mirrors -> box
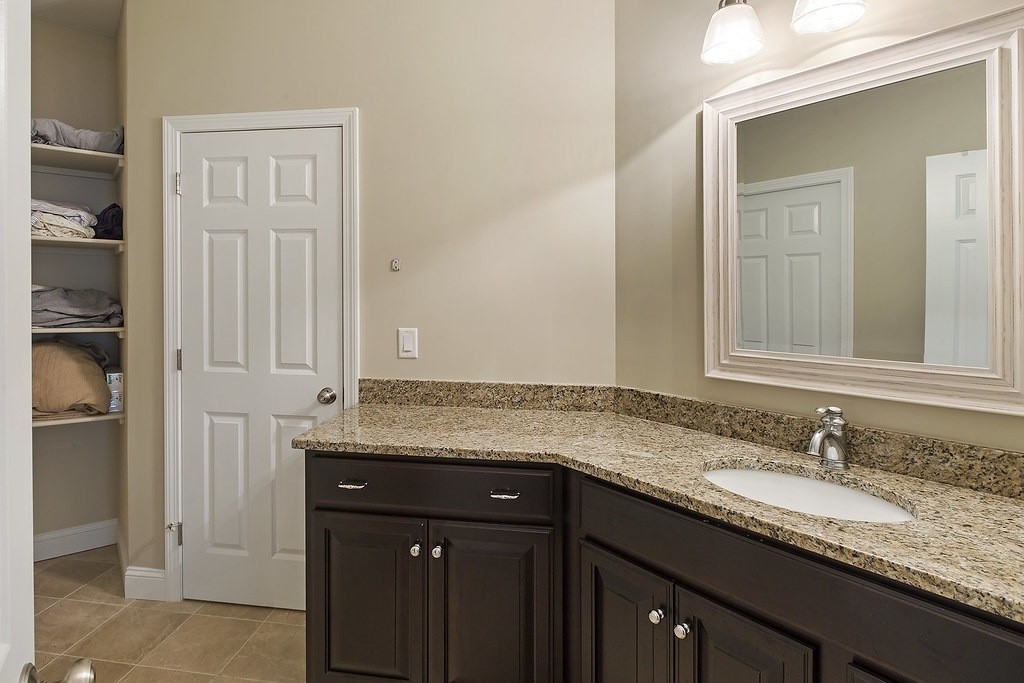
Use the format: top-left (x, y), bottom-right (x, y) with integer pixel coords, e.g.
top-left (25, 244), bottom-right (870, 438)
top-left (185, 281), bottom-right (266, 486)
top-left (702, 2), bottom-right (1024, 418)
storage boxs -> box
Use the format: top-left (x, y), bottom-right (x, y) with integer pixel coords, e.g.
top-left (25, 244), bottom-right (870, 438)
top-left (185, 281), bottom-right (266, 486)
top-left (108, 392), bottom-right (124, 412)
top-left (104, 367), bottom-right (124, 392)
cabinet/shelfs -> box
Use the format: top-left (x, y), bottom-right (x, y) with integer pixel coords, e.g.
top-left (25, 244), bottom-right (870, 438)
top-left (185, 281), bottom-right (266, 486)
top-left (32, 142), bottom-right (125, 428)
top-left (566, 470), bottom-right (1024, 683)
top-left (305, 449), bottom-right (567, 683)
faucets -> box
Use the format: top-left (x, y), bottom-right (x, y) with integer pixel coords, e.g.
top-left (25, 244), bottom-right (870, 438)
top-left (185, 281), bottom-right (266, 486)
top-left (808, 404), bottom-right (848, 471)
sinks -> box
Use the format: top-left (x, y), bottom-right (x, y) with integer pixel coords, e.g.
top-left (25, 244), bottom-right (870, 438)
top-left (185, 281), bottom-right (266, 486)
top-left (700, 460), bottom-right (919, 523)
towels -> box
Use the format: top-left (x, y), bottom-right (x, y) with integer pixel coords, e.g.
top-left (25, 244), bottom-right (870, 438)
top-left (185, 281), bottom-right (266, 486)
top-left (31, 199), bottom-right (98, 239)
top-left (31, 284), bottom-right (125, 329)
top-left (96, 203), bottom-right (122, 239)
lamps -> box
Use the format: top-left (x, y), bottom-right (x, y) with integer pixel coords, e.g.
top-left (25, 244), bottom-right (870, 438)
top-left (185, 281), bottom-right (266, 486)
top-left (700, 0), bottom-right (870, 66)
